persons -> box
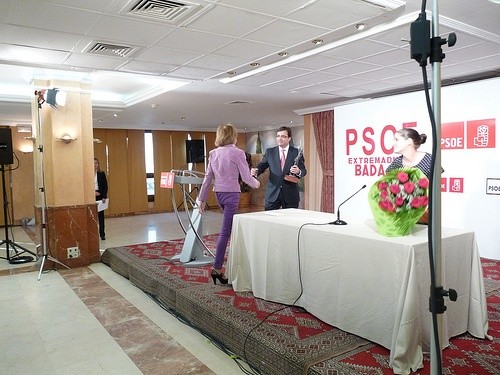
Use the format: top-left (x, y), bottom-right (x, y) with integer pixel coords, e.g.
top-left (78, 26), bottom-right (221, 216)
top-left (384, 129), bottom-right (445, 224)
top-left (198, 124), bottom-right (260, 285)
top-left (251, 126), bottom-right (307, 211)
top-left (94, 157), bottom-right (108, 240)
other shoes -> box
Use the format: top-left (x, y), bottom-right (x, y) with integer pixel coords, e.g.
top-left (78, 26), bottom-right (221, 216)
top-left (101, 236), bottom-right (105, 240)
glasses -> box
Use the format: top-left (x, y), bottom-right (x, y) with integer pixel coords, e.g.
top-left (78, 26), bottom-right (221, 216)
top-left (276, 135), bottom-right (288, 139)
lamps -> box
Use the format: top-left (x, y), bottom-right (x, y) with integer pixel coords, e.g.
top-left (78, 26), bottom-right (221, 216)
top-left (46, 88), bottom-right (66, 107)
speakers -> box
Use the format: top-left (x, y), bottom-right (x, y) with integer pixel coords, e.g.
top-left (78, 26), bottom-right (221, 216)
top-left (0, 127), bottom-right (13, 164)
top-left (186, 139), bottom-right (204, 163)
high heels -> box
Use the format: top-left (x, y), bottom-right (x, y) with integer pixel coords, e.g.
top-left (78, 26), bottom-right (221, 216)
top-left (211, 269), bottom-right (228, 285)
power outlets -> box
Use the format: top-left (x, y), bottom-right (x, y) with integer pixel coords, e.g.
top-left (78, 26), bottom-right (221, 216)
top-left (67, 247), bottom-right (78, 259)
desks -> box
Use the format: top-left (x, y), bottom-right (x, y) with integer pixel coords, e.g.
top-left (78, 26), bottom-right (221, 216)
top-left (223, 208), bottom-right (492, 375)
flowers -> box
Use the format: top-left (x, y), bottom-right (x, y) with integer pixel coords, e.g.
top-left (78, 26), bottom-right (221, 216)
top-left (377, 173), bottom-right (428, 212)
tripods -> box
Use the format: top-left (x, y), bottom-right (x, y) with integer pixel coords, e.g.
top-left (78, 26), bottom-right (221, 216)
top-left (0, 164), bottom-right (36, 260)
top-left (31, 106), bottom-right (70, 280)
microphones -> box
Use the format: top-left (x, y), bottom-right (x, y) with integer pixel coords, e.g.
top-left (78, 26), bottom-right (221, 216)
top-left (333, 185), bottom-right (366, 225)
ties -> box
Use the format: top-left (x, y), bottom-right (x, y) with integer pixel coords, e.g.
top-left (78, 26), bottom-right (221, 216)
top-left (280, 150), bottom-right (286, 172)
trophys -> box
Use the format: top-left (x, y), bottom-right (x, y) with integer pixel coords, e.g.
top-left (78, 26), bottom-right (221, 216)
top-left (284, 141), bottom-right (303, 183)
